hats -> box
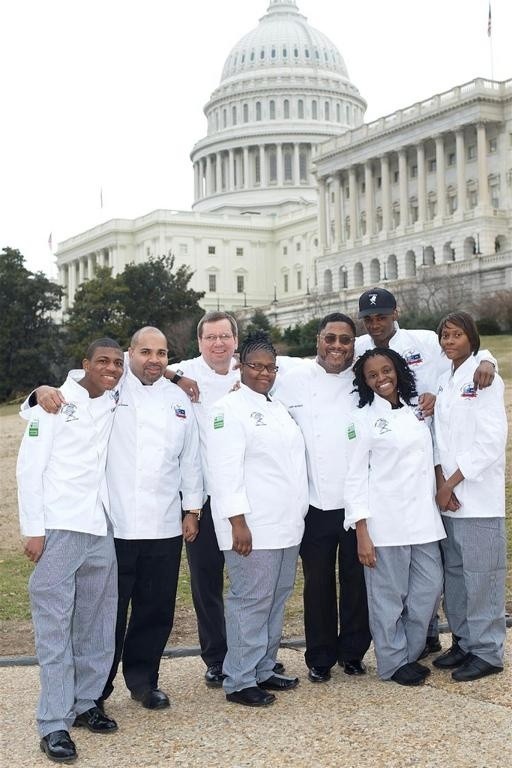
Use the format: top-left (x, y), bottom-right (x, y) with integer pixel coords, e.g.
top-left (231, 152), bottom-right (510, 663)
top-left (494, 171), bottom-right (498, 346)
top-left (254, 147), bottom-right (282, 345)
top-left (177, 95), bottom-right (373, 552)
top-left (357, 287), bottom-right (397, 319)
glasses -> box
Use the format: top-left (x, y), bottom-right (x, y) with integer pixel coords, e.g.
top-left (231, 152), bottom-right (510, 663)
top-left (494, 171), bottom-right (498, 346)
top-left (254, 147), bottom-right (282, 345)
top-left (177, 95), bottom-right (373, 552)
top-left (321, 334), bottom-right (355, 346)
top-left (200, 333), bottom-right (232, 340)
top-left (243, 362), bottom-right (279, 374)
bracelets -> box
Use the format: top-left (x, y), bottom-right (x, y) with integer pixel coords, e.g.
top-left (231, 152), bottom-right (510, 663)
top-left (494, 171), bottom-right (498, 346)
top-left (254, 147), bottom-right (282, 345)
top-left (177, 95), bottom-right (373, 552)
top-left (185, 509), bottom-right (204, 521)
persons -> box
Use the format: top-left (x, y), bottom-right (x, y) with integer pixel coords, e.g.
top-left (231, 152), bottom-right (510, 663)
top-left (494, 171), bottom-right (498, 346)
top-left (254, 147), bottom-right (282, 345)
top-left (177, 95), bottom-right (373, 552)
top-left (166, 311), bottom-right (284, 687)
top-left (341, 346), bottom-right (447, 687)
top-left (230, 312), bottom-right (437, 683)
top-left (15, 337), bottom-right (200, 762)
top-left (199, 337), bottom-right (309, 707)
top-left (353, 287), bottom-right (498, 661)
top-left (428, 310), bottom-right (508, 683)
top-left (20, 326), bottom-right (207, 710)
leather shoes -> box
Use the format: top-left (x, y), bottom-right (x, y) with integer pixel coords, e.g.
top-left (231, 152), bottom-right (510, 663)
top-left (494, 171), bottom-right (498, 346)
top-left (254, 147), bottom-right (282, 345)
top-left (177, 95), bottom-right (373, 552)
top-left (40, 730), bottom-right (78, 762)
top-left (72, 705), bottom-right (117, 735)
top-left (130, 688), bottom-right (171, 711)
top-left (391, 635), bottom-right (504, 686)
top-left (205, 662), bottom-right (299, 707)
top-left (338, 660), bottom-right (368, 677)
top-left (307, 667), bottom-right (335, 683)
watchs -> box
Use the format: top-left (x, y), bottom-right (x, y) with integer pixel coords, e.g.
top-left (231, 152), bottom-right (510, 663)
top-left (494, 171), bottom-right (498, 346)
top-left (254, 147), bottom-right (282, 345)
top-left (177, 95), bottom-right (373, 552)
top-left (170, 369), bottom-right (184, 383)
